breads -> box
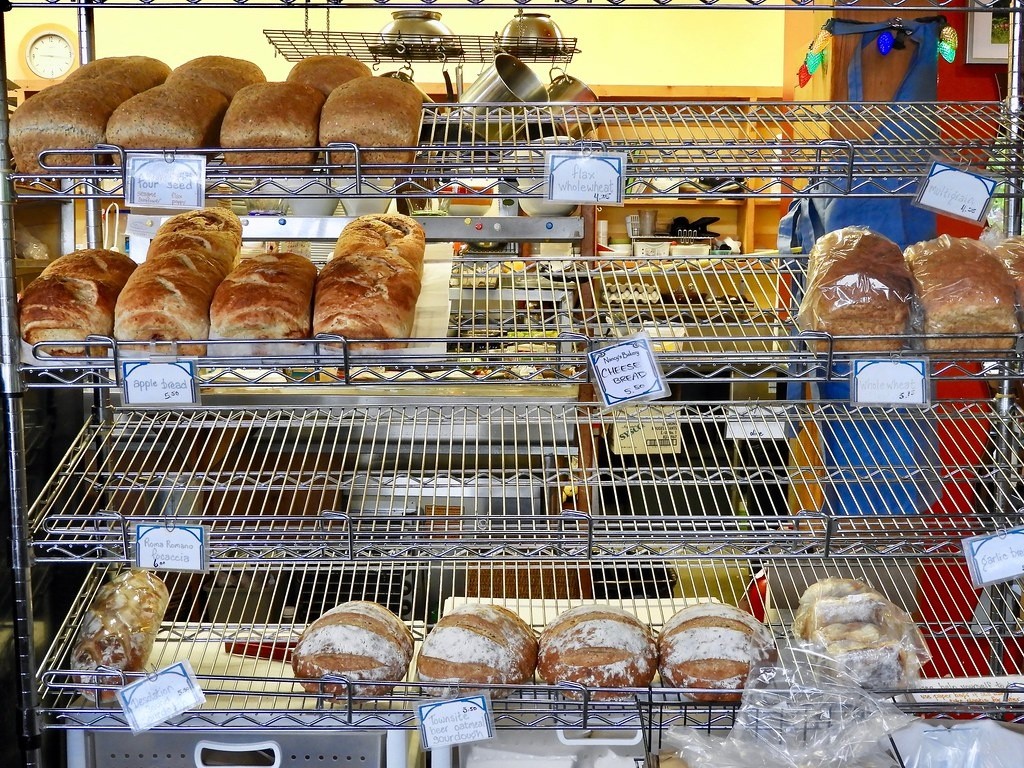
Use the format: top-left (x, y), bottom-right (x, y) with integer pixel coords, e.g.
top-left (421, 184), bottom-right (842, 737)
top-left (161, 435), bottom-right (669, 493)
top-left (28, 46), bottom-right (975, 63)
top-left (73, 568), bottom-right (171, 702)
top-left (19, 208), bottom-right (426, 364)
top-left (803, 226), bottom-right (1023, 350)
top-left (798, 581), bottom-right (916, 688)
top-left (292, 599), bottom-right (537, 700)
top-left (7, 56), bottom-right (422, 178)
top-left (538, 603), bottom-right (774, 702)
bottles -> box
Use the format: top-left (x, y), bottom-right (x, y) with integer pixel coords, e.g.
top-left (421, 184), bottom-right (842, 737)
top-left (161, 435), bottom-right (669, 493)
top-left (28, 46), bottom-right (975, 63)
top-left (217, 186), bottom-right (231, 210)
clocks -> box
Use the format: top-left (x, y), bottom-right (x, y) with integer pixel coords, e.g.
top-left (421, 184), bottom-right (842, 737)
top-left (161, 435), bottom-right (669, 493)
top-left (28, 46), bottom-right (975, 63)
top-left (18, 23), bottom-right (80, 79)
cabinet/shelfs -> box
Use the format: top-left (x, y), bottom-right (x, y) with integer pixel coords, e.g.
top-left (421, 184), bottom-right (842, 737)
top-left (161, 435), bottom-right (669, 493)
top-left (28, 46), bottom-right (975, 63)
top-left (0, 0), bottom-right (1024, 768)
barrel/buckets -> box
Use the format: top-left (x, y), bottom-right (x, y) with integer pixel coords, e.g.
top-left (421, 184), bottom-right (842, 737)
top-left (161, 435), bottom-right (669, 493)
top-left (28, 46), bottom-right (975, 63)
top-left (522, 67), bottom-right (603, 142)
top-left (458, 48), bottom-right (550, 142)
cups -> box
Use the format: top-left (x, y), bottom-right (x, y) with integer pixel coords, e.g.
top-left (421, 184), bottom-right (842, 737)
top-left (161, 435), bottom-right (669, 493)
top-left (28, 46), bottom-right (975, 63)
top-left (597, 220), bottom-right (608, 247)
top-left (638, 210), bottom-right (657, 236)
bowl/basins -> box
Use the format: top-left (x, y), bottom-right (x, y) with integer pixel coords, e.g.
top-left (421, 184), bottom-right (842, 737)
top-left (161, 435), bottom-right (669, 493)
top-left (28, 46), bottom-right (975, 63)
top-left (310, 243), bottom-right (338, 270)
top-left (240, 241), bottom-right (264, 258)
top-left (518, 197), bottom-right (578, 217)
top-left (437, 186), bottom-right (493, 216)
top-left (241, 192), bottom-right (289, 216)
top-left (498, 14), bottom-right (564, 57)
top-left (286, 188), bottom-right (340, 216)
top-left (368, 10), bottom-right (461, 60)
top-left (335, 186), bottom-right (395, 217)
top-left (402, 191), bottom-right (453, 217)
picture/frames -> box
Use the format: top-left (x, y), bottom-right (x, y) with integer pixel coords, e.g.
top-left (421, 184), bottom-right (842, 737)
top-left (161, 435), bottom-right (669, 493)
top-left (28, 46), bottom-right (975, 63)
top-left (966, 0), bottom-right (1012, 64)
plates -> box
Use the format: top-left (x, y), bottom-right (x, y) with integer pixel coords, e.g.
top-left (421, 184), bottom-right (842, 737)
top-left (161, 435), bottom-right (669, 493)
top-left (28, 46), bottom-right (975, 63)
top-left (599, 251), bottom-right (630, 258)
